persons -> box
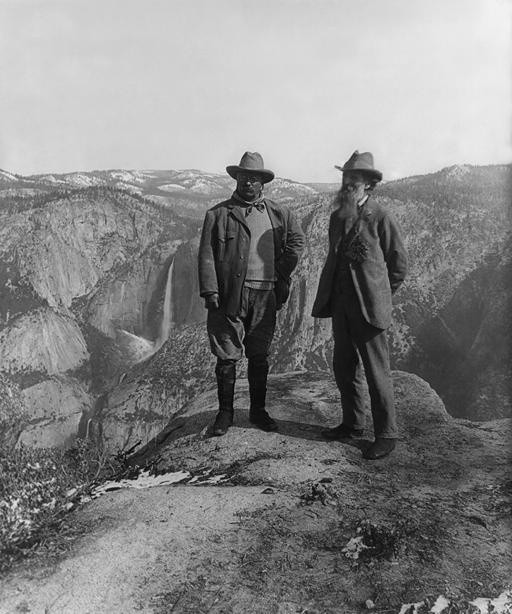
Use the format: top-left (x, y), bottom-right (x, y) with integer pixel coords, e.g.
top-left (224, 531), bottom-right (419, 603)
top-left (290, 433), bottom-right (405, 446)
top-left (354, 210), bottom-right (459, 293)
top-left (310, 150), bottom-right (409, 460)
top-left (198, 152), bottom-right (306, 436)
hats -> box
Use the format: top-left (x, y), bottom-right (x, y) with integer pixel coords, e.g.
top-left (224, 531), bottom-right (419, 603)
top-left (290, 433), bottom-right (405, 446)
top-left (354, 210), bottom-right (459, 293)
top-left (225, 152), bottom-right (274, 183)
top-left (334, 150), bottom-right (382, 183)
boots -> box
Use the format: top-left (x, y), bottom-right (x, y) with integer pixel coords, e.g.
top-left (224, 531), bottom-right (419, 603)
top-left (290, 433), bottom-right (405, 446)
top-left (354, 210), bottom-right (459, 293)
top-left (213, 364), bottom-right (236, 436)
top-left (247, 364), bottom-right (278, 431)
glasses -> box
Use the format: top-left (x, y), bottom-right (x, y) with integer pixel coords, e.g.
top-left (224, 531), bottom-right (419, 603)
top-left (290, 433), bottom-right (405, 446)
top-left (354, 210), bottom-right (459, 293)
top-left (234, 174), bottom-right (258, 183)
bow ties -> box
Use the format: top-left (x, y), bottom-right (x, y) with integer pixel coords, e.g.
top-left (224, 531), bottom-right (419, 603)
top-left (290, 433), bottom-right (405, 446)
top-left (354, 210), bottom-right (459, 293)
top-left (246, 203), bottom-right (264, 215)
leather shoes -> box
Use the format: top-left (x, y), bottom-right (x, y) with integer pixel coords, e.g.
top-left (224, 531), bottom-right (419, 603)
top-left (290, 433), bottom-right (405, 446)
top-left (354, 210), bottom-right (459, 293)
top-left (321, 423), bottom-right (366, 439)
top-left (362, 439), bottom-right (395, 459)
